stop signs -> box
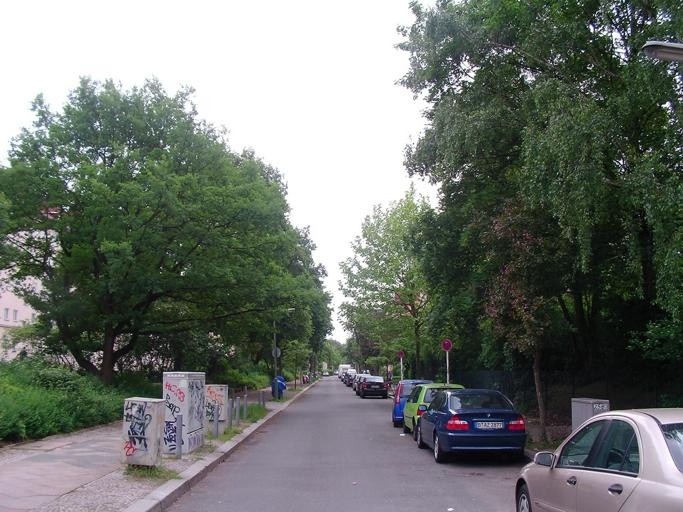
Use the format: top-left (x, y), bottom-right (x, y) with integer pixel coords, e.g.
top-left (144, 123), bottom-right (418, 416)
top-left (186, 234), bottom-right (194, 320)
top-left (442, 339), bottom-right (452, 352)
top-left (399, 351), bottom-right (404, 358)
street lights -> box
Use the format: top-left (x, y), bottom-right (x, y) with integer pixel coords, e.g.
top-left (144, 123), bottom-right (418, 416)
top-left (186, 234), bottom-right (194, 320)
top-left (273, 307), bottom-right (296, 401)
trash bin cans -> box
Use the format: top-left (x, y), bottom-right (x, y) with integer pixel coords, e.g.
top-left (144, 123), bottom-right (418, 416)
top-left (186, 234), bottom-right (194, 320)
top-left (272, 376), bottom-right (286, 399)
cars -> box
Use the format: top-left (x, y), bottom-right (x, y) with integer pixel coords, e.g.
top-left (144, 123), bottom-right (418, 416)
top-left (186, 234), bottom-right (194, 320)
top-left (337, 363), bottom-right (389, 399)
top-left (390, 378), bottom-right (530, 462)
top-left (511, 404), bottom-right (683, 512)
